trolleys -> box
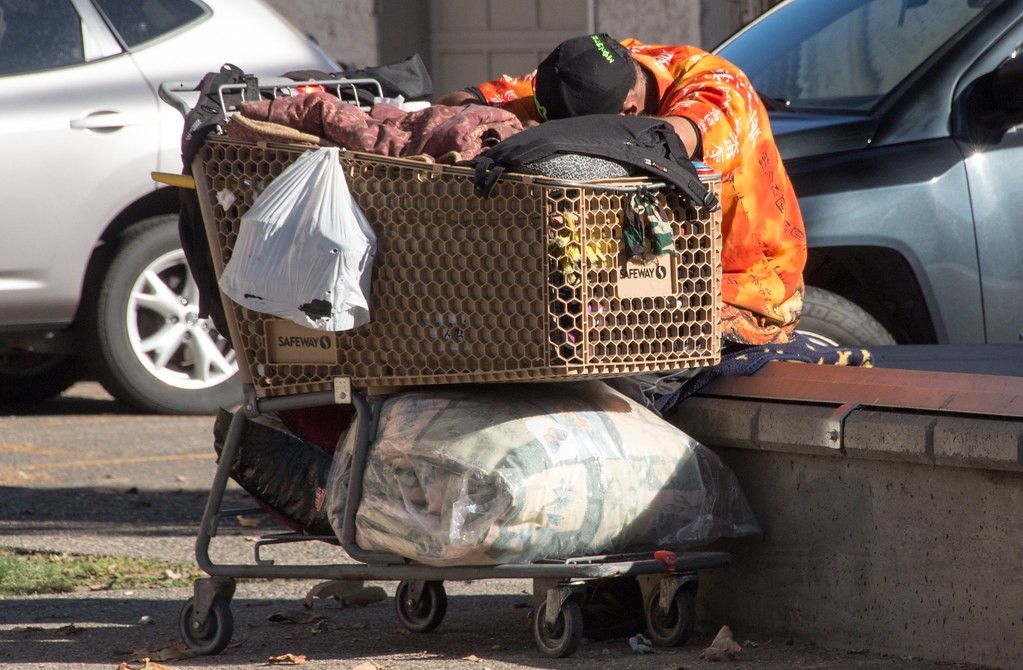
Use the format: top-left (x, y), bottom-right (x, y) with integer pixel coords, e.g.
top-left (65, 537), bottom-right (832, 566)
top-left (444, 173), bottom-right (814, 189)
top-left (157, 67), bottom-right (740, 660)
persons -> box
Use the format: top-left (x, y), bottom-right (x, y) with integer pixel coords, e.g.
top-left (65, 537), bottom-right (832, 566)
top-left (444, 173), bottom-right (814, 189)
top-left (440, 34), bottom-right (809, 628)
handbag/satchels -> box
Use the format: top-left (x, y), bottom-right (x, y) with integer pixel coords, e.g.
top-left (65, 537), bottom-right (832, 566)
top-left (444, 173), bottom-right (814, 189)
top-left (217, 147), bottom-right (376, 332)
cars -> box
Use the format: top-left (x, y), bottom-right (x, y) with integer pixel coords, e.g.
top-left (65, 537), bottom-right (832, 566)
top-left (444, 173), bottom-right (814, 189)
top-left (704, 0), bottom-right (1023, 356)
top-left (0, 0), bottom-right (353, 419)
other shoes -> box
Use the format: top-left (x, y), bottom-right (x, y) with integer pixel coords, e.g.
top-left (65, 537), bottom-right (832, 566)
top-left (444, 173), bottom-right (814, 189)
top-left (556, 575), bottom-right (647, 639)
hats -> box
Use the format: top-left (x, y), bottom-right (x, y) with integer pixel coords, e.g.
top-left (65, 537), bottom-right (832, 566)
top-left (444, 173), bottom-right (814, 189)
top-left (531, 34), bottom-right (635, 124)
